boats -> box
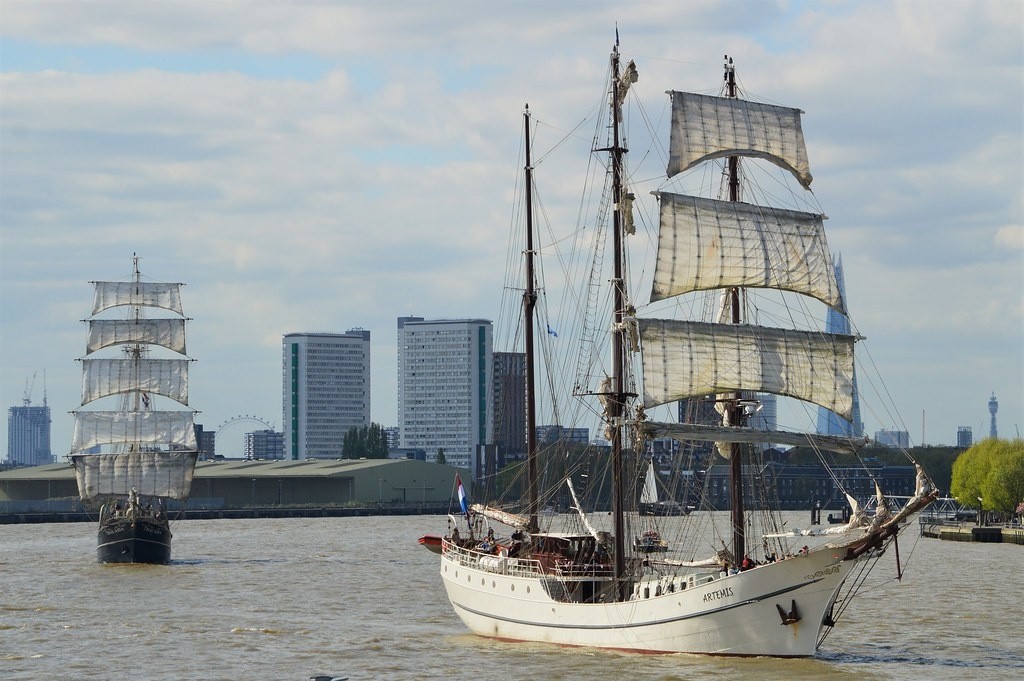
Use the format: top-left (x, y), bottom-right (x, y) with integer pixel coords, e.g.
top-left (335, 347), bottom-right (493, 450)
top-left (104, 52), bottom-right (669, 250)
top-left (418, 535), bottom-right (483, 556)
top-left (632, 529), bottom-right (669, 554)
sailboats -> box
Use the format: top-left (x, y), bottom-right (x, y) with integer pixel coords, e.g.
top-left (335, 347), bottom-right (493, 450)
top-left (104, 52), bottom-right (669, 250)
top-left (637, 457), bottom-right (695, 516)
top-left (439, 21), bottom-right (942, 660)
top-left (59, 252), bottom-right (209, 570)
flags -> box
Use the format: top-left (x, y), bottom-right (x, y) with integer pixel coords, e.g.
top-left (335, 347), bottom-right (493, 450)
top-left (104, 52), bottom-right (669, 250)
top-left (463, 485), bottom-right (464, 486)
top-left (548, 324), bottom-right (558, 337)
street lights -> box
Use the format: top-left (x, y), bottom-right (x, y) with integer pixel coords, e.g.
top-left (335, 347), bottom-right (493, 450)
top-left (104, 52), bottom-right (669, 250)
top-left (979, 498), bottom-right (983, 528)
top-left (955, 496), bottom-right (959, 527)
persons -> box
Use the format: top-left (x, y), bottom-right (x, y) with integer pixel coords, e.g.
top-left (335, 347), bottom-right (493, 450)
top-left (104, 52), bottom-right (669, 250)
top-left (488, 526), bottom-right (494, 540)
top-left (455, 474), bottom-right (470, 516)
top-left (722, 553), bottom-right (755, 576)
top-left (452, 528), bottom-right (459, 541)
top-left (765, 552), bottom-right (775, 563)
